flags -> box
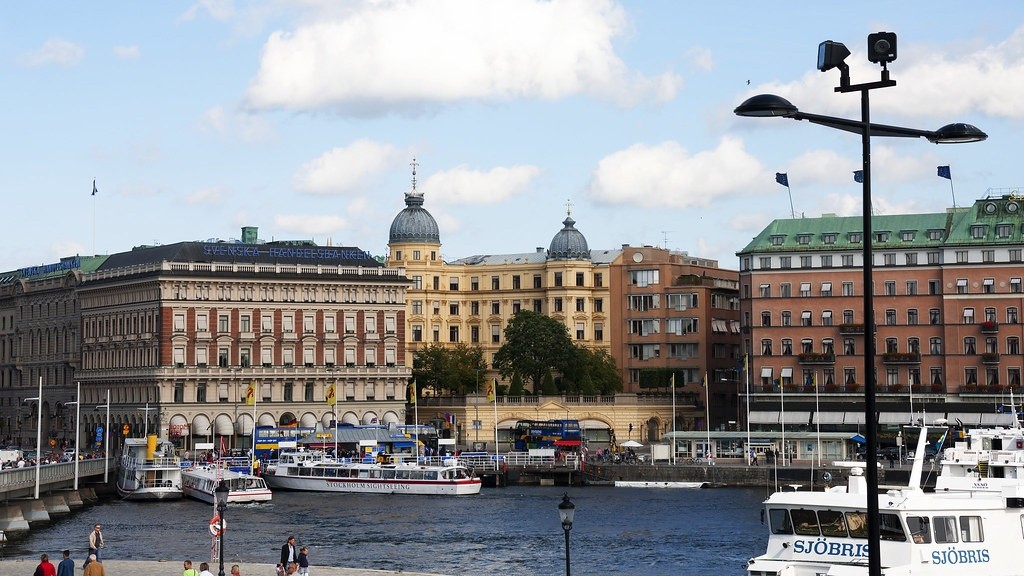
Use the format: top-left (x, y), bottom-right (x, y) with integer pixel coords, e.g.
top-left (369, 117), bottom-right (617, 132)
top-left (937, 166), bottom-right (950, 179)
top-left (328, 382), bottom-right (336, 405)
top-left (92, 181), bottom-right (97, 195)
top-left (775, 174), bottom-right (789, 188)
top-left (409, 382), bottom-right (415, 404)
top-left (486, 381), bottom-right (495, 402)
top-left (246, 382), bottom-right (255, 405)
top-left (854, 170), bottom-right (863, 182)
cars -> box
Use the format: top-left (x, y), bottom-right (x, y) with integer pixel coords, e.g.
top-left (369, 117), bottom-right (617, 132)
top-left (856, 446), bottom-right (905, 460)
top-left (63, 448), bottom-right (83, 456)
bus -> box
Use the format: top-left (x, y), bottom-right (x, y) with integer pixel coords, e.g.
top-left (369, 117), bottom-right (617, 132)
top-left (252, 425), bottom-right (316, 458)
top-left (510, 419), bottom-right (581, 455)
top-left (323, 423), bottom-right (439, 462)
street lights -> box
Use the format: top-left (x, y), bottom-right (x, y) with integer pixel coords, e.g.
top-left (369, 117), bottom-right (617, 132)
top-left (96, 390), bottom-right (110, 483)
top-left (733, 31), bottom-right (989, 576)
top-left (24, 376), bottom-right (43, 499)
top-left (214, 478), bottom-right (230, 576)
top-left (64, 382), bottom-right (80, 490)
top-left (558, 491), bottom-right (575, 576)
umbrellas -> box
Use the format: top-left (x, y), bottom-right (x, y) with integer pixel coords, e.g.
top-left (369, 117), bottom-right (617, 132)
top-left (618, 441), bottom-right (644, 449)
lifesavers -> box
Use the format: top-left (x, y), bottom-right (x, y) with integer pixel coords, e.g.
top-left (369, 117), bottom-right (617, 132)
top-left (210, 517), bottom-right (227, 536)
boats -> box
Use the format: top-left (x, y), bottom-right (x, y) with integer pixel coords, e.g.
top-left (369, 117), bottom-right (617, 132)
top-left (117, 403), bottom-right (184, 500)
top-left (933, 387), bottom-right (1024, 492)
top-left (260, 450), bottom-right (482, 494)
top-left (182, 463), bottom-right (272, 504)
top-left (747, 409), bottom-right (1024, 576)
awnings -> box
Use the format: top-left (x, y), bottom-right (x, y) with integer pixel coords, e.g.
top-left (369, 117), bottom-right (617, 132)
top-left (760, 283), bottom-right (832, 378)
top-left (958, 278), bottom-right (1021, 317)
top-left (748, 411), bottom-right (1014, 432)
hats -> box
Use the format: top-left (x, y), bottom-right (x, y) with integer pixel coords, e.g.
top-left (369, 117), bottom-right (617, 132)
top-left (89, 554), bottom-right (96, 560)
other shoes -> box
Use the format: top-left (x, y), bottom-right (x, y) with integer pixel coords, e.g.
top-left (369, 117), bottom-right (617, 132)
top-left (83, 564), bottom-right (86, 569)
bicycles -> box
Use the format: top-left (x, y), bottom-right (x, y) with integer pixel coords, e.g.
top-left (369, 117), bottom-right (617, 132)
top-left (686, 456), bottom-right (703, 465)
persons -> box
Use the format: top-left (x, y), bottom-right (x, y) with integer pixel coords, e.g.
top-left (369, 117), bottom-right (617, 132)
top-left (456, 448), bottom-right (469, 456)
top-left (889, 454), bottom-right (894, 468)
top-left (83, 524), bottom-right (104, 568)
top-left (199, 445), bottom-right (232, 461)
top-left (276, 537), bottom-right (309, 576)
top-left (751, 447), bottom-right (779, 466)
top-left (558, 447), bottom-right (609, 464)
top-left (425, 447), bottom-right (446, 456)
top-left (198, 563), bottom-right (214, 576)
top-left (248, 447), bottom-right (267, 475)
top-left (57, 550), bottom-right (74, 576)
top-left (83, 554), bottom-right (105, 576)
top-left (231, 566), bottom-right (240, 576)
top-left (183, 560), bottom-right (198, 576)
top-left (0, 450), bottom-right (106, 471)
top-left (33, 554), bottom-right (56, 576)
top-left (327, 448), bottom-right (364, 462)
top-left (183, 450), bottom-right (189, 461)
